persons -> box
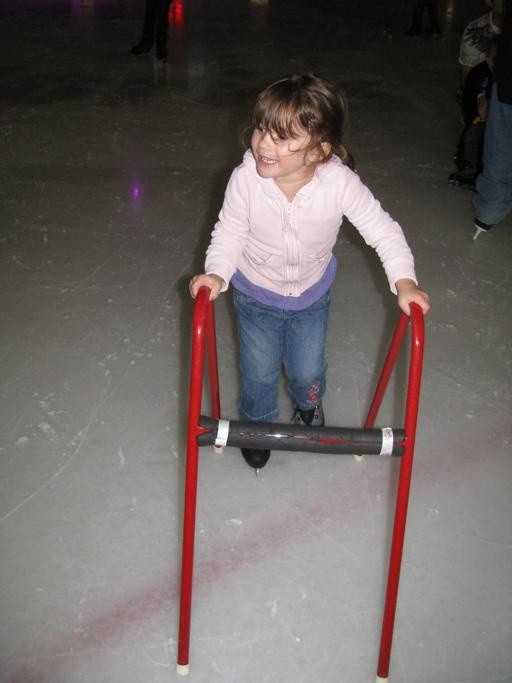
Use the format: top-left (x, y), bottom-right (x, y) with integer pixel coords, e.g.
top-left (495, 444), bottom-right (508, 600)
top-left (458, 13), bottom-right (509, 243)
top-left (188, 74), bottom-right (431, 469)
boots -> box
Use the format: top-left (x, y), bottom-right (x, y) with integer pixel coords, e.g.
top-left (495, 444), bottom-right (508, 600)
top-left (291, 401), bottom-right (324, 426)
top-left (242, 449), bottom-right (271, 468)
top-left (406, 26), bottom-right (440, 36)
top-left (475, 219), bottom-right (494, 232)
top-left (449, 172), bottom-right (475, 192)
top-left (132, 38), bottom-right (167, 59)
